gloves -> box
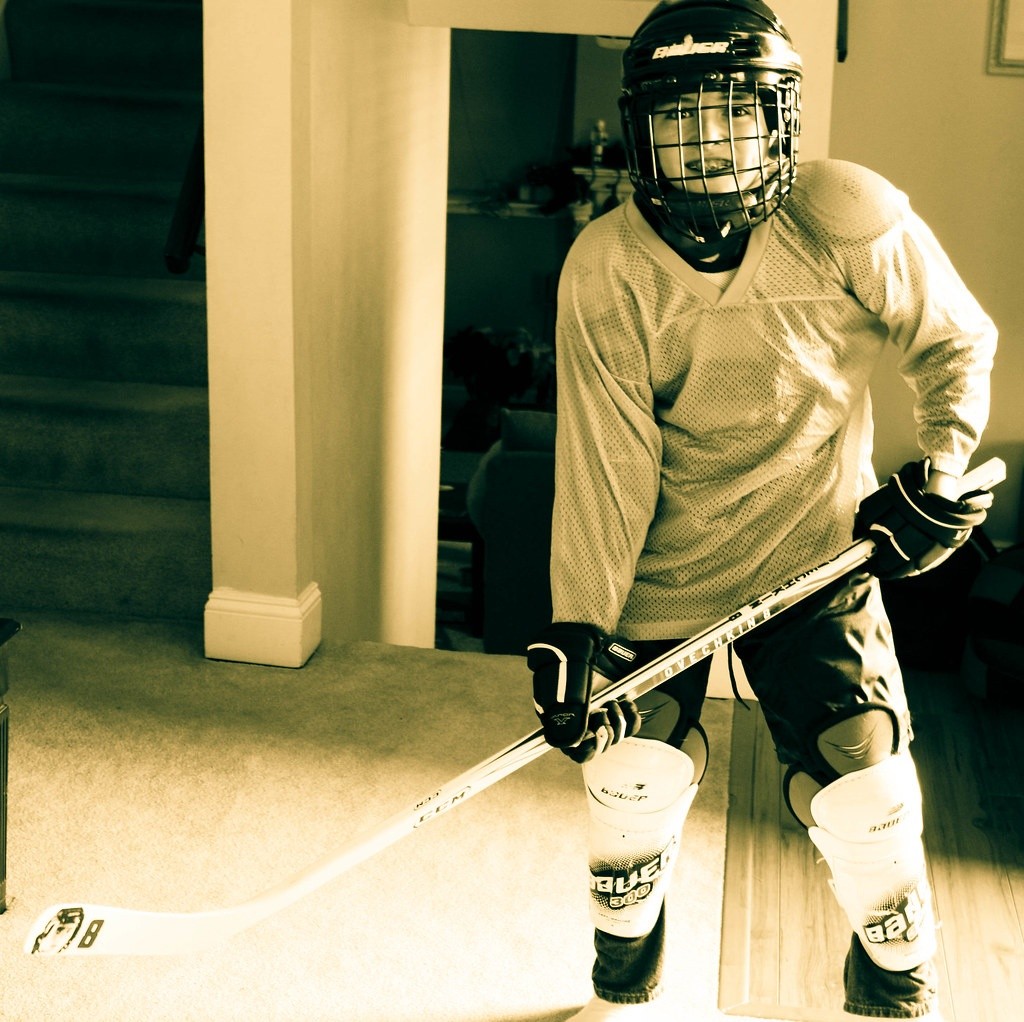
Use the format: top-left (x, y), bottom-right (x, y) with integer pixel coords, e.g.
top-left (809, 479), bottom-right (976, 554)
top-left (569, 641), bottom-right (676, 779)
top-left (852, 458), bottom-right (995, 579)
top-left (526, 622), bottom-right (642, 765)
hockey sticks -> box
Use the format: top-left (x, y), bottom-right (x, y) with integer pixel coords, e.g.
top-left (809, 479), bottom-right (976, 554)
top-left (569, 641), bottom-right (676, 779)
top-left (22, 454), bottom-right (1008, 966)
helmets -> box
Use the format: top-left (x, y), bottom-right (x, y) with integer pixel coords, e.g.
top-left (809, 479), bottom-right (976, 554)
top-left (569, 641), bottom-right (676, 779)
top-left (618, 0), bottom-right (804, 243)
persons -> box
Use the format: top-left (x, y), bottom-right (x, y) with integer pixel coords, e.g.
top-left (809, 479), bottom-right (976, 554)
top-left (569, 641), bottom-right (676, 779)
top-left (526, 0), bottom-right (999, 1022)
top-left (590, 120), bottom-right (609, 163)
